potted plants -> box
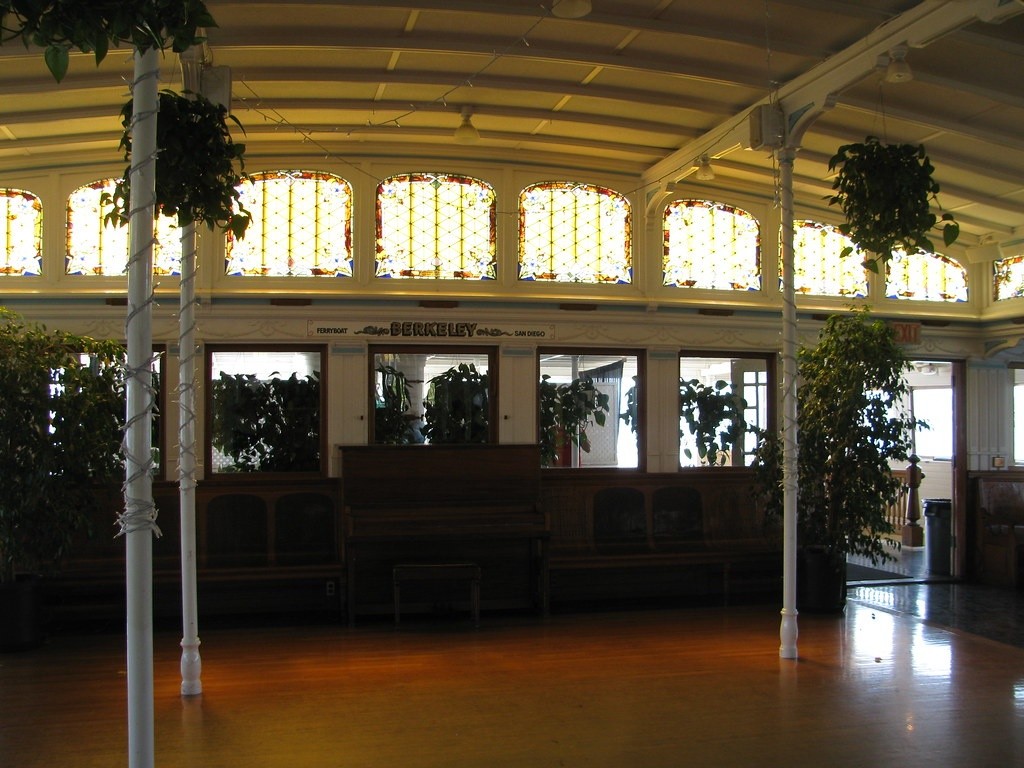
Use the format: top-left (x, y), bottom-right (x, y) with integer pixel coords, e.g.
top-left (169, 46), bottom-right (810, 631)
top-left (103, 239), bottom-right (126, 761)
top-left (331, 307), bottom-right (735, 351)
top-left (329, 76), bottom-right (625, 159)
top-left (679, 377), bottom-right (748, 465)
top-left (741, 309), bottom-right (931, 620)
top-left (821, 134), bottom-right (960, 276)
top-left (0, 0), bottom-right (222, 86)
top-left (421, 363), bottom-right (490, 446)
top-left (539, 375), bottom-right (611, 467)
top-left (373, 360), bottom-right (424, 446)
top-left (211, 370), bottom-right (270, 473)
top-left (99, 89), bottom-right (252, 239)
top-left (271, 371), bottom-right (321, 472)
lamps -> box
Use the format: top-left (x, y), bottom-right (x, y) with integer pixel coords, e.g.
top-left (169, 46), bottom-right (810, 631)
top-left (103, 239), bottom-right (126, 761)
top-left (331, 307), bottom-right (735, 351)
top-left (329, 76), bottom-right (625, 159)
top-left (888, 45), bottom-right (913, 85)
top-left (453, 106), bottom-right (480, 144)
top-left (553, 0), bottom-right (593, 19)
top-left (697, 155), bottom-right (715, 181)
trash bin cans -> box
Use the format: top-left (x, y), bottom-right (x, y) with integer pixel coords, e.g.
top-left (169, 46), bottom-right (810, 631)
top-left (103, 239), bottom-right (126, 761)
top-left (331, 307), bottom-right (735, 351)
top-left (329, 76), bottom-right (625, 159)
top-left (924, 498), bottom-right (951, 573)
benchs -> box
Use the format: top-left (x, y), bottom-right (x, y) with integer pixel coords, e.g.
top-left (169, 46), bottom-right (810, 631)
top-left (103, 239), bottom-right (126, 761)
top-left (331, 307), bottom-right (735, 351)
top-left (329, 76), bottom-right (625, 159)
top-left (974, 475), bottom-right (1024, 588)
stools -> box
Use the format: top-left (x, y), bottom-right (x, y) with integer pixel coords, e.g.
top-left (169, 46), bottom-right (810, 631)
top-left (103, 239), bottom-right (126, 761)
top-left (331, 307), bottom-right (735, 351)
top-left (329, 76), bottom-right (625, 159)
top-left (386, 558), bottom-right (480, 630)
